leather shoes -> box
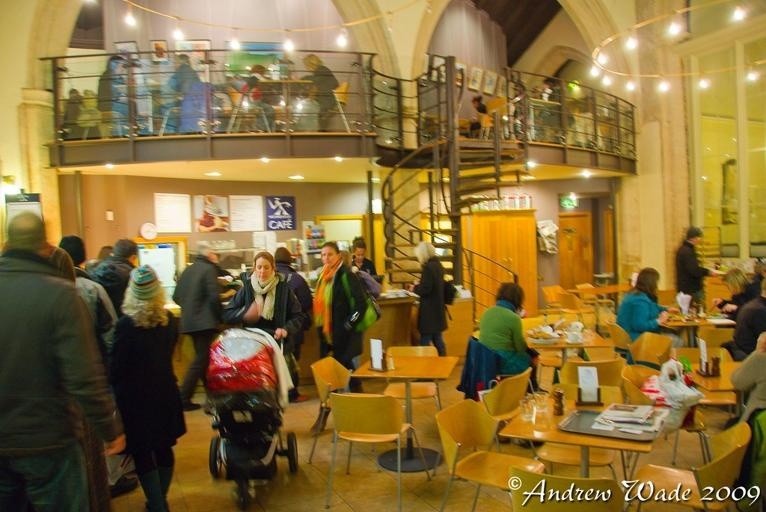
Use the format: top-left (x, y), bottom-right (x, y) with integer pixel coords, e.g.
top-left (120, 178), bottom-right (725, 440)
top-left (182, 402), bottom-right (202, 411)
top-left (290, 394), bottom-right (308, 402)
top-left (109, 476), bottom-right (137, 497)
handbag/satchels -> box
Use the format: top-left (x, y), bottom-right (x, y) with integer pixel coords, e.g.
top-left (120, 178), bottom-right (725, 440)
top-left (352, 298), bottom-right (377, 332)
top-left (285, 352), bottom-right (300, 375)
top-left (637, 359), bottom-right (704, 433)
top-left (444, 279), bottom-right (456, 306)
top-left (478, 380), bottom-right (500, 402)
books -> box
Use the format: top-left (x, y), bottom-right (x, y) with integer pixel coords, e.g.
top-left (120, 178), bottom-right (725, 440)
top-left (591, 403), bottom-right (670, 434)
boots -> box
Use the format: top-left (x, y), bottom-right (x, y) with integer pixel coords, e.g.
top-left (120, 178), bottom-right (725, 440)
top-left (351, 384), bottom-right (364, 393)
top-left (310, 407), bottom-right (330, 435)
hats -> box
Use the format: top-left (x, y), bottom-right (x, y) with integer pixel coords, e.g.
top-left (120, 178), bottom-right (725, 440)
top-left (58, 235), bottom-right (86, 265)
top-left (129, 264), bottom-right (160, 301)
top-left (275, 248), bottom-right (290, 261)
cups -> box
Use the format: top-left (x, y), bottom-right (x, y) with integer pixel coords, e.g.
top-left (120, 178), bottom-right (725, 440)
top-left (533, 391), bottom-right (548, 412)
top-left (519, 399), bottom-right (537, 422)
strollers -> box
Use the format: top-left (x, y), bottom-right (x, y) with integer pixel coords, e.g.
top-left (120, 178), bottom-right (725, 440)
top-left (198, 325), bottom-right (299, 505)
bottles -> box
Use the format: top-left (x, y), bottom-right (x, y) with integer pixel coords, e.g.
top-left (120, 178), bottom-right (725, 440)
top-left (239, 264), bottom-right (248, 284)
top-left (552, 388), bottom-right (563, 415)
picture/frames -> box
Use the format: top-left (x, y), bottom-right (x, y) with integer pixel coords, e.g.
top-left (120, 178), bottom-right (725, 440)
top-left (150, 39), bottom-right (169, 64)
top-left (174, 38), bottom-right (212, 84)
top-left (113, 41), bottom-right (141, 61)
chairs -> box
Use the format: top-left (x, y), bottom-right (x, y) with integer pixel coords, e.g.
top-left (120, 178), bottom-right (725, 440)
top-left (384, 345), bottom-right (444, 420)
top-left (224, 86), bottom-right (270, 133)
top-left (506, 466), bottom-right (629, 512)
top-left (620, 363), bottom-right (712, 468)
top-left (73, 88), bottom-right (123, 140)
top-left (625, 331), bottom-right (673, 367)
top-left (308, 355), bottom-right (356, 473)
top-left (537, 382), bottom-right (625, 480)
top-left (671, 345), bottom-right (740, 416)
top-left (472, 95), bottom-right (511, 138)
top-left (328, 78), bottom-right (350, 133)
top-left (434, 398), bottom-right (541, 511)
top-left (625, 420), bottom-right (751, 512)
top-left (576, 281), bottom-right (592, 290)
top-left (561, 356), bottom-right (627, 392)
top-left (483, 367), bottom-right (539, 422)
top-left (556, 292), bottom-right (595, 328)
top-left (157, 101), bottom-right (186, 138)
top-left (461, 337), bottom-right (535, 403)
top-left (326, 390), bottom-right (433, 512)
top-left (541, 284), bottom-right (571, 323)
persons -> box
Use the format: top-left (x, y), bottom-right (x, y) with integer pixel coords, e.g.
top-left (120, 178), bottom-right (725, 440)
top-left (734, 278), bottom-right (766, 355)
top-left (210, 252), bottom-right (237, 302)
top-left (309, 241), bottom-right (367, 436)
top-left (408, 242), bottom-right (453, 383)
top-left (725, 332), bottom-right (766, 501)
top-left (105, 264), bottom-right (186, 511)
top-left (64, 50), bottom-right (576, 147)
top-left (59, 236), bottom-right (137, 500)
top-left (614, 267), bottom-right (685, 371)
top-left (32, 243), bottom-right (110, 511)
top-left (751, 256), bottom-right (766, 282)
top-left (84, 239), bottom-right (138, 320)
top-left (479, 282), bottom-right (544, 447)
top-left (711, 268), bottom-right (760, 362)
top-left (222, 252), bottom-right (304, 356)
top-left (350, 237), bottom-right (379, 299)
top-left (84, 246), bottom-right (114, 273)
top-left (0, 210), bottom-right (127, 511)
top-left (240, 247), bottom-right (312, 402)
top-left (171, 241), bottom-right (227, 412)
top-left (335, 251), bottom-right (381, 356)
top-left (675, 227), bottom-right (717, 347)
top-left (197, 195), bottom-right (229, 232)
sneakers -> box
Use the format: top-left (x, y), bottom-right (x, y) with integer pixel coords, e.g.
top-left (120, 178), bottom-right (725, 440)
top-left (496, 436), bottom-right (512, 445)
top-left (518, 440), bottom-right (544, 448)
